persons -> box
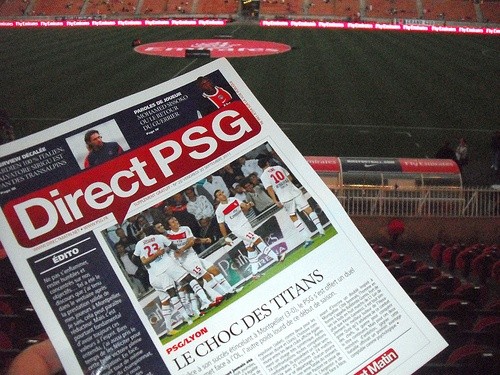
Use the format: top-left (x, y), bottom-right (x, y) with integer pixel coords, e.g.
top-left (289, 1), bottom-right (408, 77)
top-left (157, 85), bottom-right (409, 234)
top-left (193, 76), bottom-right (239, 120)
top-left (101, 147), bottom-right (327, 337)
top-left (6, 338), bottom-right (64, 375)
top-left (83, 129), bottom-right (125, 170)
top-left (0, 0), bottom-right (495, 25)
top-left (435, 129), bottom-right (500, 174)
top-left (387, 214), bottom-right (405, 252)
top-left (131, 38), bottom-right (142, 46)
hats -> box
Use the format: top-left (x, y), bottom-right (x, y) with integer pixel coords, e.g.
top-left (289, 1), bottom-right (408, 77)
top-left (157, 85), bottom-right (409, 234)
top-left (232, 183), bottom-right (240, 189)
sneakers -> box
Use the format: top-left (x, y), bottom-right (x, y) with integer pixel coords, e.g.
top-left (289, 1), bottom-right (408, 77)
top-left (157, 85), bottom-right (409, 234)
top-left (167, 330), bottom-right (180, 336)
top-left (280, 253), bottom-right (286, 262)
top-left (304, 241), bottom-right (315, 248)
top-left (182, 291), bottom-right (237, 325)
top-left (319, 234), bottom-right (325, 239)
top-left (253, 272), bottom-right (265, 280)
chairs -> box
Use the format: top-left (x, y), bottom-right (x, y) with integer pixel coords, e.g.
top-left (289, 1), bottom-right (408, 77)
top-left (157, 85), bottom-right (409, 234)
top-left (370, 239), bottom-right (500, 375)
top-left (0, 256), bottom-right (49, 375)
